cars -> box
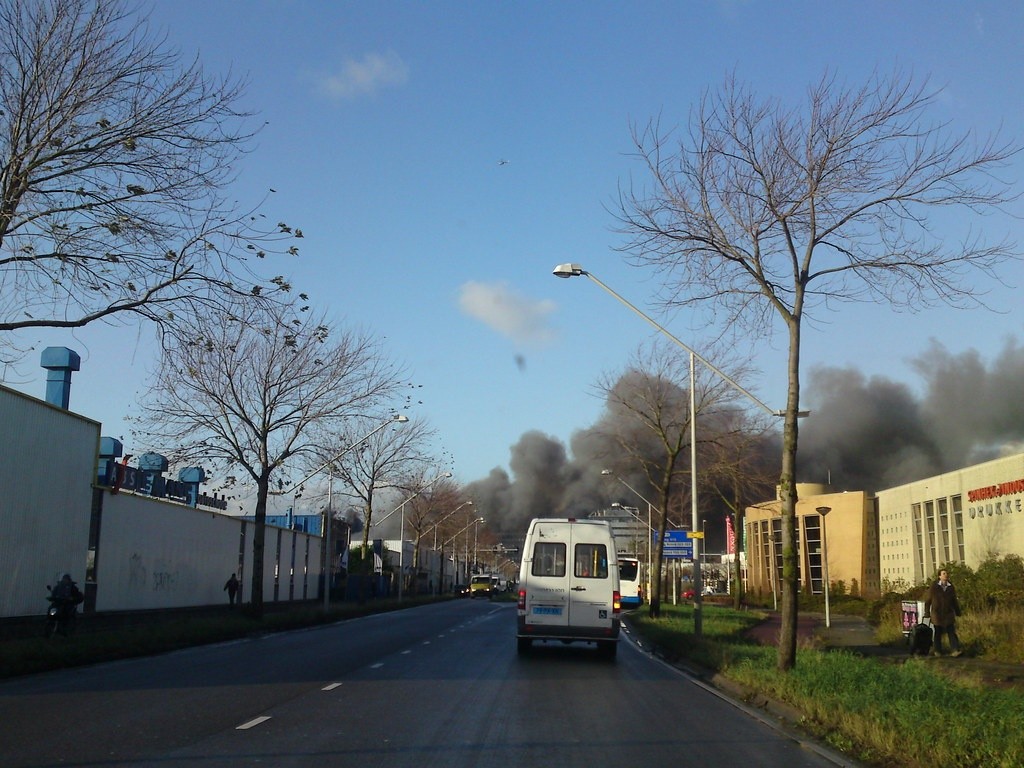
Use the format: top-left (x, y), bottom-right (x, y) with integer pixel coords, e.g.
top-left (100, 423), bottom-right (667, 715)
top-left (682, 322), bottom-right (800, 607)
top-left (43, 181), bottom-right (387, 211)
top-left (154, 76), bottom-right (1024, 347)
top-left (682, 586), bottom-right (724, 599)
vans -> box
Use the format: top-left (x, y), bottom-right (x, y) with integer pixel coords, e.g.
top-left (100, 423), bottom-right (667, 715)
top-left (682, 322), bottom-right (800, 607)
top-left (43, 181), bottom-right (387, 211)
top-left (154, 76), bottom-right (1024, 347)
top-left (517, 516), bottom-right (622, 661)
top-left (459, 575), bottom-right (509, 598)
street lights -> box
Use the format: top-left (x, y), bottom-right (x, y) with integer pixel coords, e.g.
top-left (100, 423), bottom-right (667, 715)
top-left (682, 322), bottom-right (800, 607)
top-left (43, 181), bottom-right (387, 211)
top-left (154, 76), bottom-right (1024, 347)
top-left (598, 467), bottom-right (685, 599)
top-left (766, 532), bottom-right (780, 612)
top-left (418, 499), bottom-right (490, 598)
top-left (273, 414), bottom-right (408, 610)
top-left (387, 472), bottom-right (454, 604)
top-left (815, 504), bottom-right (832, 629)
top-left (551, 260), bottom-right (778, 636)
top-left (611, 502), bottom-right (660, 606)
top-left (702, 518), bottom-right (708, 588)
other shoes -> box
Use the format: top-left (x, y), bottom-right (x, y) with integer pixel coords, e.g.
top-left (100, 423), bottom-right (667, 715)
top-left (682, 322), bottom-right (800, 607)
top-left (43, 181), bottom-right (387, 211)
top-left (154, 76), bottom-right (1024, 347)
top-left (934, 651), bottom-right (941, 657)
top-left (950, 650), bottom-right (962, 657)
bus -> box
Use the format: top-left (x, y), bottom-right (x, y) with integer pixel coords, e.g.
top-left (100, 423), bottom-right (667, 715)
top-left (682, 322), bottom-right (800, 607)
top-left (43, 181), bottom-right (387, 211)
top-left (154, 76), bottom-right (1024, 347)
top-left (618, 557), bottom-right (643, 610)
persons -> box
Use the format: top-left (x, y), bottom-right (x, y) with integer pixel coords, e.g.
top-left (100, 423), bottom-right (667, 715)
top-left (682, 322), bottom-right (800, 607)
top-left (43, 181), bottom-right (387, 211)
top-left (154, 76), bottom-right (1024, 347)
top-left (924, 568), bottom-right (963, 657)
top-left (44, 573), bottom-right (84, 622)
top-left (506, 578), bottom-right (514, 593)
top-left (407, 566), bottom-right (421, 598)
top-left (392, 570), bottom-right (405, 598)
top-left (335, 566), bottom-right (348, 584)
top-left (223, 573), bottom-right (239, 604)
top-left (382, 565), bottom-right (391, 598)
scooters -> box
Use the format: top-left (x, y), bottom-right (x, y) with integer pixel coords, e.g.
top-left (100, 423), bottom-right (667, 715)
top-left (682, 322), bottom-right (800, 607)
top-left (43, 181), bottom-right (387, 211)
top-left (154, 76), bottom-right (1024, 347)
top-left (44, 581), bottom-right (82, 636)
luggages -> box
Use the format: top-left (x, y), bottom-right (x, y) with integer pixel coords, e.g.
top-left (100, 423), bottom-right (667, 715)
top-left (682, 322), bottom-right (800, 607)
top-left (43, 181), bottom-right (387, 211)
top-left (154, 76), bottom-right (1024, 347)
top-left (907, 616), bottom-right (933, 656)
